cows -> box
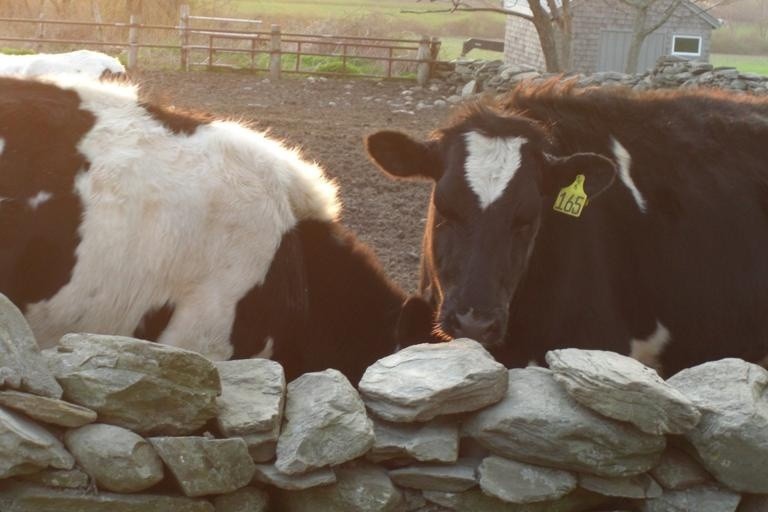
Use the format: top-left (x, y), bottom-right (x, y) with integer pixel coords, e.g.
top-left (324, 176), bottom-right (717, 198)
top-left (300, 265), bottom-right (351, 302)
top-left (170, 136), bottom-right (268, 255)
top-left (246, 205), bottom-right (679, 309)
top-left (1, 70), bottom-right (453, 389)
top-left (367, 82), bottom-right (766, 380)
